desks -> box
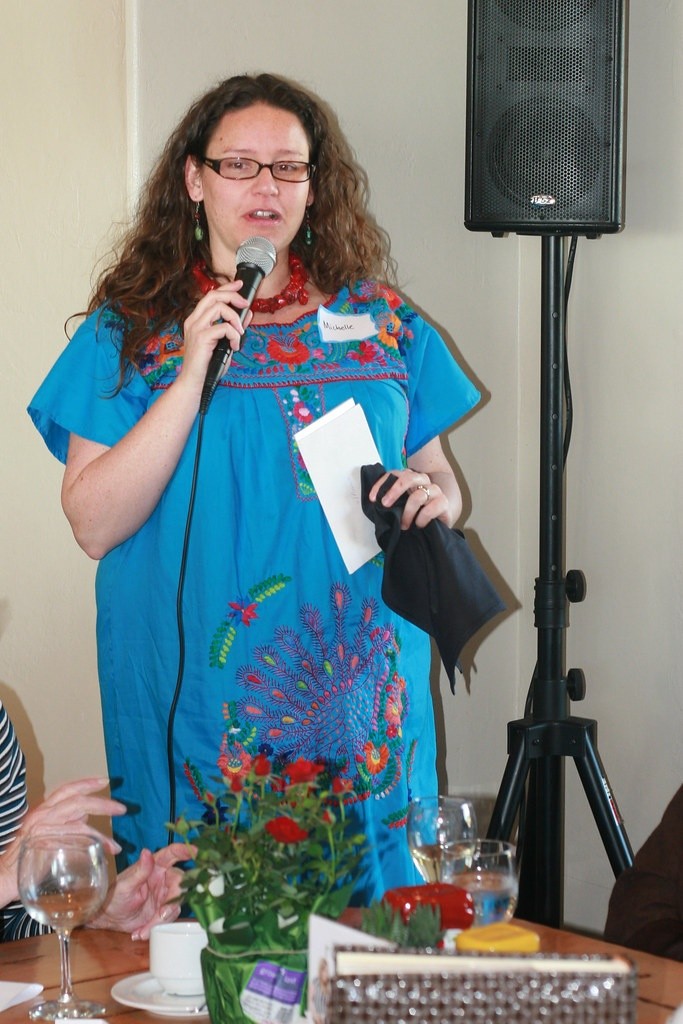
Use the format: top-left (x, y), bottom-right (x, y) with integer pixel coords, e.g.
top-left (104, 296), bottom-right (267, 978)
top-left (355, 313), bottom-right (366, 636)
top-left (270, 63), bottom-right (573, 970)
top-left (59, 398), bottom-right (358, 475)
top-left (0, 907), bottom-right (683, 1024)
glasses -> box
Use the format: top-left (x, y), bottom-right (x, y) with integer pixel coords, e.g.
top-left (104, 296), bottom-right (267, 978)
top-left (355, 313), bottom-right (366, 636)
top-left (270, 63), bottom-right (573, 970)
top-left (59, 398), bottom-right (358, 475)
top-left (201, 156), bottom-right (316, 182)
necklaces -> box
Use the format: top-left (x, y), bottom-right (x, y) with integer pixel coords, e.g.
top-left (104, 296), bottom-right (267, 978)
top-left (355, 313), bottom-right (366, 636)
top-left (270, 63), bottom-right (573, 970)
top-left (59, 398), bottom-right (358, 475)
top-left (193, 253), bottom-right (309, 314)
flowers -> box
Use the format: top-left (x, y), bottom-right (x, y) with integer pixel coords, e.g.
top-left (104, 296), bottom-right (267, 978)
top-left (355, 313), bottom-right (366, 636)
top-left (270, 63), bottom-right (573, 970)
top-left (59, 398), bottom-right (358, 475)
top-left (161, 750), bottom-right (368, 1024)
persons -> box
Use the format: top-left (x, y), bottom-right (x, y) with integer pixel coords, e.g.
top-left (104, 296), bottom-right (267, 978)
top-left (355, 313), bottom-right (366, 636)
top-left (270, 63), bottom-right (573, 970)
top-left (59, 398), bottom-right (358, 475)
top-left (0, 697), bottom-right (199, 943)
top-left (26, 73), bottom-right (483, 910)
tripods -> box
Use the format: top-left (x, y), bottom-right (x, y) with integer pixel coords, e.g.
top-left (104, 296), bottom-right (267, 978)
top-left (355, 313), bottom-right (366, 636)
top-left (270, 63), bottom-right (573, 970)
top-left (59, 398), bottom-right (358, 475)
top-left (476, 234), bottom-right (633, 932)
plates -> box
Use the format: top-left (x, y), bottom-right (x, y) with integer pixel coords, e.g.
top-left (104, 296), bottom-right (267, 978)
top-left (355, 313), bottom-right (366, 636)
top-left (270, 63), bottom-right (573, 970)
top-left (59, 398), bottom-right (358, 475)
top-left (109, 974), bottom-right (212, 1020)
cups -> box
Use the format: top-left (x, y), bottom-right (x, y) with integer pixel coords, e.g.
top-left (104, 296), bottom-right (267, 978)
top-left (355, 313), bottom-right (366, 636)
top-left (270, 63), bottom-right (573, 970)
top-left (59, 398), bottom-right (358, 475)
top-left (440, 839), bottom-right (519, 926)
top-left (149, 922), bottom-right (208, 995)
top-left (406, 794), bottom-right (480, 884)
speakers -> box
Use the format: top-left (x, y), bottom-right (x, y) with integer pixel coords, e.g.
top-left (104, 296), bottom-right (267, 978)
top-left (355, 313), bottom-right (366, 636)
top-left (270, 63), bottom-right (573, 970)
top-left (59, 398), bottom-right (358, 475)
top-left (464, 0), bottom-right (630, 240)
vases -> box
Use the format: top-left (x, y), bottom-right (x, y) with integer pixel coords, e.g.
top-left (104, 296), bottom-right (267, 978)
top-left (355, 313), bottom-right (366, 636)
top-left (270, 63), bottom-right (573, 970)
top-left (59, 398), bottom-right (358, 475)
top-left (200, 945), bottom-right (308, 1024)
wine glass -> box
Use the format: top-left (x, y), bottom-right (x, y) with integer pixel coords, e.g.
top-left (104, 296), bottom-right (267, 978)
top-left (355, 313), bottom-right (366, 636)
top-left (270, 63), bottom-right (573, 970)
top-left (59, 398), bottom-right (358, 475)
top-left (16, 835), bottom-right (105, 1023)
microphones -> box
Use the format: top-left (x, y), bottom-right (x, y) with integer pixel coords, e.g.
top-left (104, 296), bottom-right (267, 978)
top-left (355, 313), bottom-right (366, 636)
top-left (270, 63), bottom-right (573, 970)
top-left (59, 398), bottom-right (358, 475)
top-left (199, 236), bottom-right (277, 415)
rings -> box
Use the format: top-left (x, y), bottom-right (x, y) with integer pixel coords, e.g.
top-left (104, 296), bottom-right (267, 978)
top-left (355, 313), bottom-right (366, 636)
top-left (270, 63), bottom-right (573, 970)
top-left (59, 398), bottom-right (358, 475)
top-left (417, 485), bottom-right (429, 500)
